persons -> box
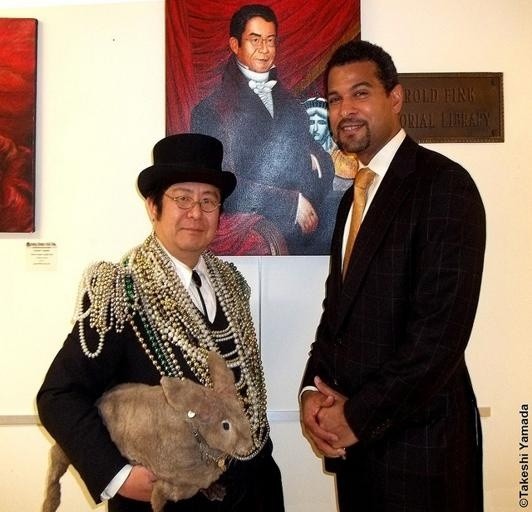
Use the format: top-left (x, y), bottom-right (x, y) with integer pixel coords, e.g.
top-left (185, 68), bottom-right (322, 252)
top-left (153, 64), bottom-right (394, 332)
top-left (35, 135), bottom-right (285, 512)
top-left (298, 40), bottom-right (485, 511)
top-left (190, 4), bottom-right (346, 254)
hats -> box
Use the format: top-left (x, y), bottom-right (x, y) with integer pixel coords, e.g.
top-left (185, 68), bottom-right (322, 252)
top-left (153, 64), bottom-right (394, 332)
top-left (137, 134), bottom-right (237, 198)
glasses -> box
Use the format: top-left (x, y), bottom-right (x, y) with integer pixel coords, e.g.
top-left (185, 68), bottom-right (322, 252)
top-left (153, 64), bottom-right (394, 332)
top-left (164, 194), bottom-right (221, 213)
top-left (241, 36), bottom-right (278, 48)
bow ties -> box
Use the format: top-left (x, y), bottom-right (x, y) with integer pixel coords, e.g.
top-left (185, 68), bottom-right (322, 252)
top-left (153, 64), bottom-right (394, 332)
top-left (248, 79), bottom-right (277, 94)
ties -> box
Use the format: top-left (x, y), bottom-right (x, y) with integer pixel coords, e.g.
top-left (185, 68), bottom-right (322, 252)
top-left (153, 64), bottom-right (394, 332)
top-left (342, 167), bottom-right (376, 278)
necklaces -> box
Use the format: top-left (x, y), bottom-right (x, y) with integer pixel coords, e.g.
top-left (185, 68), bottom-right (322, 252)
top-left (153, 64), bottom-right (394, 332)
top-left (67, 231), bottom-right (270, 463)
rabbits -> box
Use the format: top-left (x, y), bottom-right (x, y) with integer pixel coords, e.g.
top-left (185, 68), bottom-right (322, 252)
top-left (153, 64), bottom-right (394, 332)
top-left (42, 351), bottom-right (255, 511)
